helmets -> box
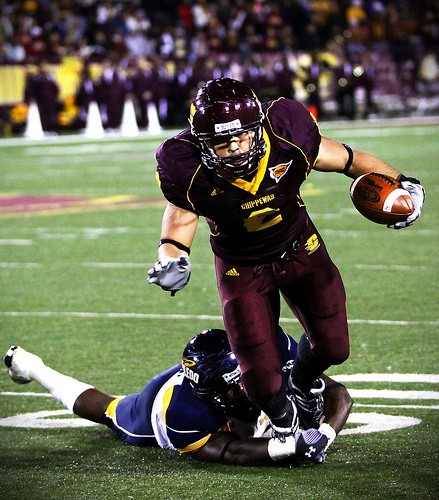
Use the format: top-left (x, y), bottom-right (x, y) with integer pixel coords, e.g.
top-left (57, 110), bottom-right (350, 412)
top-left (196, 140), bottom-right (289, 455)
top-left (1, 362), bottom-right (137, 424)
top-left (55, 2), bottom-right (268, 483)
top-left (180, 328), bottom-right (253, 418)
top-left (186, 78), bottom-right (267, 178)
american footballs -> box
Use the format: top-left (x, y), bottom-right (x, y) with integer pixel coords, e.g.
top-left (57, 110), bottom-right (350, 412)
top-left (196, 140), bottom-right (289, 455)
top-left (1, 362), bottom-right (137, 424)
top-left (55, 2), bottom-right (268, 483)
top-left (350, 172), bottom-right (415, 225)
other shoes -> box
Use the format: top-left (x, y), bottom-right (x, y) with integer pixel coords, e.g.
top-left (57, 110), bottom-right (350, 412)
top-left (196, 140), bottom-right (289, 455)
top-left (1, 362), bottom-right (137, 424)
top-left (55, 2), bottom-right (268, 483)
top-left (268, 395), bottom-right (299, 467)
top-left (3, 345), bottom-right (34, 384)
top-left (287, 375), bottom-right (329, 465)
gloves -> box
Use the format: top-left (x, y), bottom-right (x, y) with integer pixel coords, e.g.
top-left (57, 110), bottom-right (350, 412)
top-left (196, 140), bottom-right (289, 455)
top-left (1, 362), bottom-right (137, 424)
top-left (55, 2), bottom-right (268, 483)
top-left (313, 422), bottom-right (336, 460)
top-left (147, 255), bottom-right (192, 297)
top-left (267, 434), bottom-right (314, 464)
top-left (386, 175), bottom-right (426, 230)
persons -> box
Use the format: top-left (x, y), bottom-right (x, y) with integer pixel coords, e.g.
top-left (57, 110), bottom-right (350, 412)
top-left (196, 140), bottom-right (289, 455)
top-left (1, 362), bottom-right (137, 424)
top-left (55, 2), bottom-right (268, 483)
top-left (1, 0), bottom-right (439, 137)
top-left (145, 78), bottom-right (427, 464)
top-left (4, 325), bottom-right (354, 467)
